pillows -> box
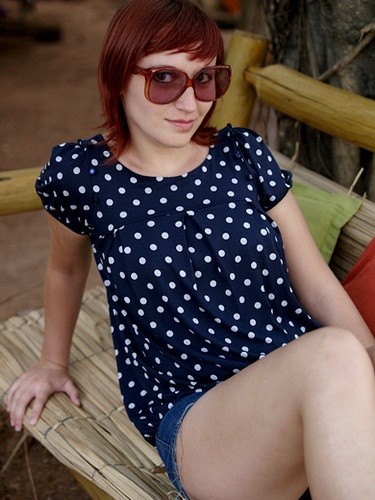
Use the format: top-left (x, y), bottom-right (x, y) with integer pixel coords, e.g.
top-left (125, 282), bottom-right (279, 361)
top-left (342, 237), bottom-right (374, 339)
top-left (292, 180), bottom-right (361, 264)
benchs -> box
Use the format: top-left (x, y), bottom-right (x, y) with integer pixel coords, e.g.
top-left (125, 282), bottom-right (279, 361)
top-left (1, 29), bottom-right (374, 500)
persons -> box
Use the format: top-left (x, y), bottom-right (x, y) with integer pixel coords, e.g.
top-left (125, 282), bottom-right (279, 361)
top-left (4, 0), bottom-right (375, 500)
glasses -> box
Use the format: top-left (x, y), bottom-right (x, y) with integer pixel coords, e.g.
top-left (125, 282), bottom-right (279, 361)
top-left (128, 63), bottom-right (232, 105)
